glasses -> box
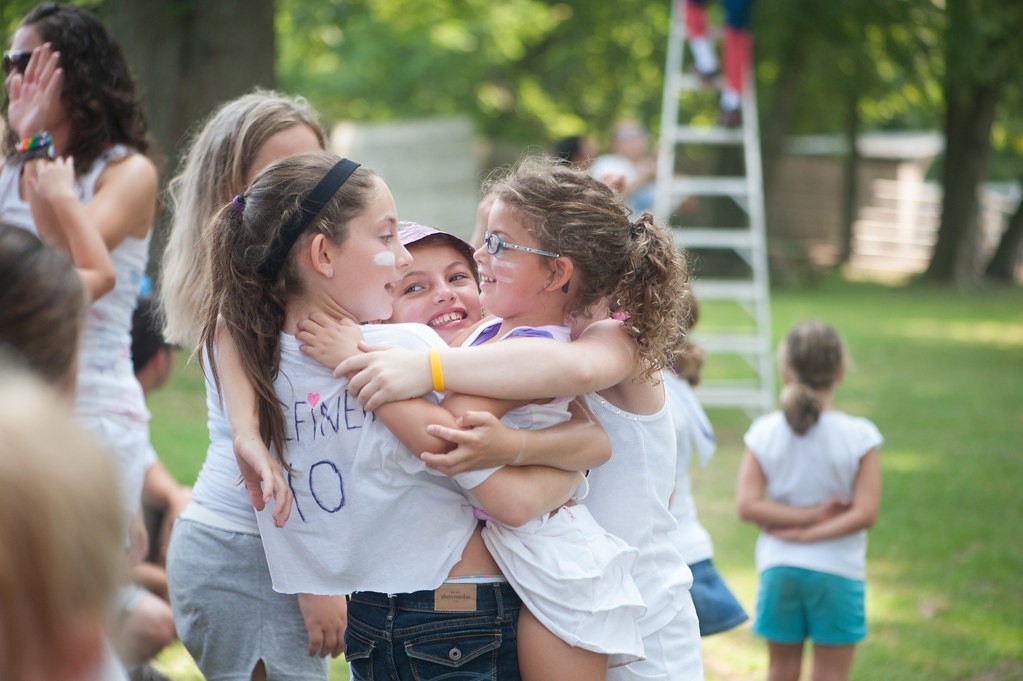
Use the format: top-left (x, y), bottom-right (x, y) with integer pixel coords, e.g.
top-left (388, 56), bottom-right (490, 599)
top-left (3, 51), bottom-right (32, 74)
top-left (484, 231), bottom-right (570, 293)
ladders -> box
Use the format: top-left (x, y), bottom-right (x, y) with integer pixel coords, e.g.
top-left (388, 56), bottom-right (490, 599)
top-left (652, 1), bottom-right (777, 420)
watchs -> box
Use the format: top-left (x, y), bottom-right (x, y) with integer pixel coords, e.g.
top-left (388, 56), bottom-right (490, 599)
top-left (20, 144), bottom-right (64, 162)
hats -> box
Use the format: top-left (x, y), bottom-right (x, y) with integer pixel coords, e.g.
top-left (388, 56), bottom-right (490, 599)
top-left (131, 298), bottom-right (166, 350)
top-left (396, 221), bottom-right (476, 263)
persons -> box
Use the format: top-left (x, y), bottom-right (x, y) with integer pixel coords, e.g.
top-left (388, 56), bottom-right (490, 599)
top-left (0, 0), bottom-right (752, 680)
top-left (749, 322), bottom-right (884, 680)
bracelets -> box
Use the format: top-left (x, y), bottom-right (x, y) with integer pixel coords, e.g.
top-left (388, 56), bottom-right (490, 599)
top-left (15, 131), bottom-right (53, 153)
top-left (430, 350), bottom-right (445, 392)
